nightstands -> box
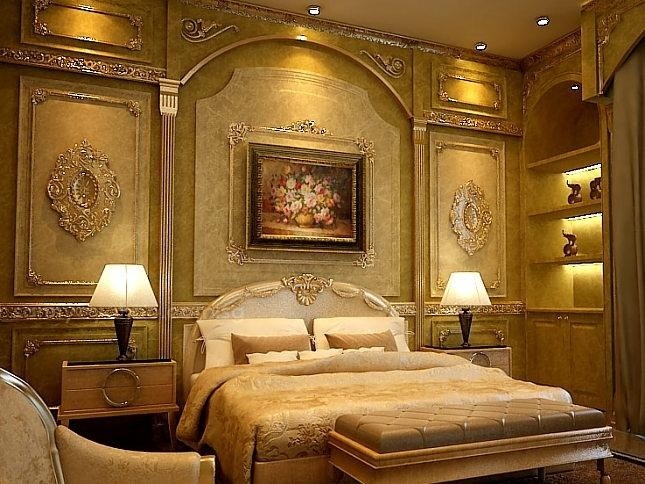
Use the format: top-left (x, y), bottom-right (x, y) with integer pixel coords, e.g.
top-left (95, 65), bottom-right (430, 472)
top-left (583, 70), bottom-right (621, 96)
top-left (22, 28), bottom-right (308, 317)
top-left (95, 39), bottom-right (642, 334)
top-left (419, 347), bottom-right (513, 392)
top-left (57, 359), bottom-right (182, 451)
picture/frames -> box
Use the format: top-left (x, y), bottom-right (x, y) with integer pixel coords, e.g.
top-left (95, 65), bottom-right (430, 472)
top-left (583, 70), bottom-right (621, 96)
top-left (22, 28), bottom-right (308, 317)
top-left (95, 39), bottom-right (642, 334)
top-left (245, 142), bottom-right (368, 253)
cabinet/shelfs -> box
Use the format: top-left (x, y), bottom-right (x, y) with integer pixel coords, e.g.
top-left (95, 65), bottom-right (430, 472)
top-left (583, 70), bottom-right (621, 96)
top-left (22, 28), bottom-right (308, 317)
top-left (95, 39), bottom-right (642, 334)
top-left (527, 142), bottom-right (605, 315)
top-left (525, 308), bottom-right (604, 425)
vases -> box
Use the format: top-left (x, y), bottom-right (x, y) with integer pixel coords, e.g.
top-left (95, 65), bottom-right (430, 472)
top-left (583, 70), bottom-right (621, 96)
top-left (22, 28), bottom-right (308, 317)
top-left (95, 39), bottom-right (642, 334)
top-left (294, 212), bottom-right (314, 228)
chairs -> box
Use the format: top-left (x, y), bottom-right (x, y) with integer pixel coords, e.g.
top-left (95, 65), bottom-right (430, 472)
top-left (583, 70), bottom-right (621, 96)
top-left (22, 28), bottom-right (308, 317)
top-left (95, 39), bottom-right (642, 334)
top-left (0, 367), bottom-right (216, 484)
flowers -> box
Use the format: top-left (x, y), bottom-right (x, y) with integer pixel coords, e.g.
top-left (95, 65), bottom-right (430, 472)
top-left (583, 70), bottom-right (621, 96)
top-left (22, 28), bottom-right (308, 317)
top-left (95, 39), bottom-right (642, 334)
top-left (272, 165), bottom-right (342, 229)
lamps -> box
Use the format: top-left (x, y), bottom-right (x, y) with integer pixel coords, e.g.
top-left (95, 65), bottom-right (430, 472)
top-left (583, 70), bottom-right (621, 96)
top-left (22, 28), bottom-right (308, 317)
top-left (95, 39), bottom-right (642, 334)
top-left (88, 263), bottom-right (159, 361)
top-left (440, 272), bottom-right (493, 349)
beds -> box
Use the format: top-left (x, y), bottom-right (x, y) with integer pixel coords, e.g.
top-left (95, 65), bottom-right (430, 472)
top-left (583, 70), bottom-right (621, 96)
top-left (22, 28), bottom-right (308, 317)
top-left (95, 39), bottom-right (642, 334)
top-left (176, 274), bottom-right (573, 484)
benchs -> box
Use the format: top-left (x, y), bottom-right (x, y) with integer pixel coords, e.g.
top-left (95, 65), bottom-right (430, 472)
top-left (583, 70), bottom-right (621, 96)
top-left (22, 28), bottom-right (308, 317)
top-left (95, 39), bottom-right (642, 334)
top-left (328, 397), bottom-right (614, 484)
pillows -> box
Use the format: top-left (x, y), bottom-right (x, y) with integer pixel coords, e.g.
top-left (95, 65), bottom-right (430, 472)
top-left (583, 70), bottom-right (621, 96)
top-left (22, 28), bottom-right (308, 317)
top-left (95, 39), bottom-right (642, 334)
top-left (195, 316), bottom-right (411, 370)
